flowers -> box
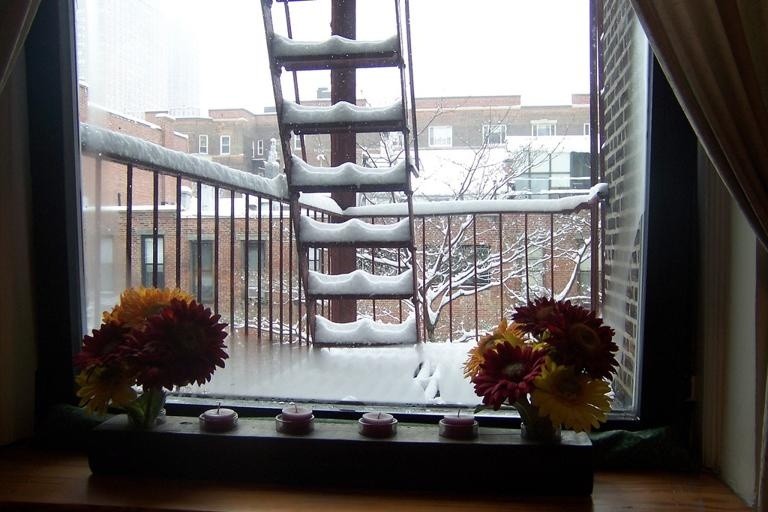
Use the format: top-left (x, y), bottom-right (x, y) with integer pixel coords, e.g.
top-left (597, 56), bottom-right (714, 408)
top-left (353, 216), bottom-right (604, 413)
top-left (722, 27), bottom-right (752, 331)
top-left (467, 293), bottom-right (622, 432)
top-left (74, 284), bottom-right (227, 423)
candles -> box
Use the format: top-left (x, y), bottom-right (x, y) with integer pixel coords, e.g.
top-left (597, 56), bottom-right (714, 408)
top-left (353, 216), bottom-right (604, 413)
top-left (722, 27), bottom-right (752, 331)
top-left (281, 402), bottom-right (312, 433)
top-left (204, 406), bottom-right (233, 431)
top-left (363, 410), bottom-right (393, 439)
top-left (444, 408), bottom-right (476, 439)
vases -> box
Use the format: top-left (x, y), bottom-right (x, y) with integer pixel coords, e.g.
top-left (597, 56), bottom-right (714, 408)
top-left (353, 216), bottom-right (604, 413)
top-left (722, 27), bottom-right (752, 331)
top-left (128, 405), bottom-right (167, 428)
top-left (521, 422), bottom-right (561, 443)
